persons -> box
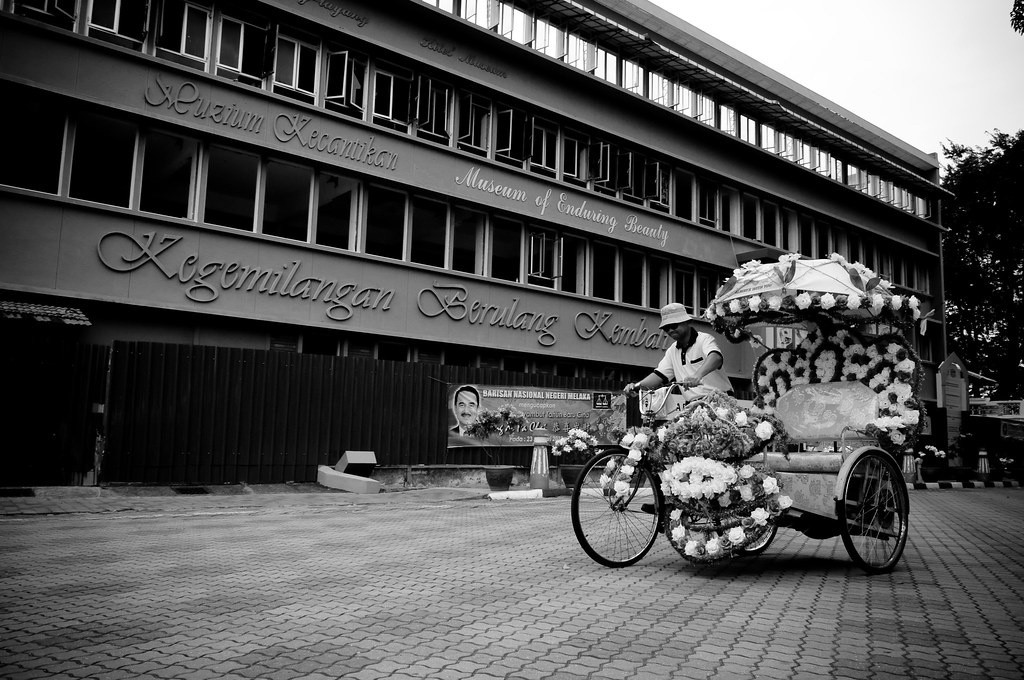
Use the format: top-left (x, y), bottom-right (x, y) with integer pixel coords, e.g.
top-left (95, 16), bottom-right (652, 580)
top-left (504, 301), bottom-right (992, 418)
top-left (623, 303), bottom-right (735, 514)
top-left (450, 386), bottom-right (480, 436)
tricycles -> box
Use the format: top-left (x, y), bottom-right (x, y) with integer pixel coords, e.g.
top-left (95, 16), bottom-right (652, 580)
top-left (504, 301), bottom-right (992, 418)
top-left (570, 379), bottom-right (911, 574)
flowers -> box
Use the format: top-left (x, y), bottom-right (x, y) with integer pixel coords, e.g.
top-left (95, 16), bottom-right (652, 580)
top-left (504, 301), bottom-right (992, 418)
top-left (597, 251), bottom-right (946, 566)
top-left (552, 427), bottom-right (606, 464)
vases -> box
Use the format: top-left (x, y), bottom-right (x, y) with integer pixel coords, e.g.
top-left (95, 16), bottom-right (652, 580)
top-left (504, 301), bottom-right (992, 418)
top-left (561, 464), bottom-right (586, 488)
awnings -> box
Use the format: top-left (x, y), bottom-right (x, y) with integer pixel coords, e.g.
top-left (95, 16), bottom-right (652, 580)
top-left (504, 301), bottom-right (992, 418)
top-left (0, 300), bottom-right (91, 327)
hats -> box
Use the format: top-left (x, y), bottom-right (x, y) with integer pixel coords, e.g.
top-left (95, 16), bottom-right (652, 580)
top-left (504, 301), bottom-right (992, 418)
top-left (658, 303), bottom-right (694, 328)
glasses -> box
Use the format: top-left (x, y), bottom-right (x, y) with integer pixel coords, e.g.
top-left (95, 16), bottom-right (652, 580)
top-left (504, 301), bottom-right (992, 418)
top-left (663, 323), bottom-right (679, 332)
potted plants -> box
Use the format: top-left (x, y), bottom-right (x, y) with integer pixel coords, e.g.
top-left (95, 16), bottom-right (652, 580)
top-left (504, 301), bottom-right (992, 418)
top-left (466, 402), bottom-right (530, 491)
